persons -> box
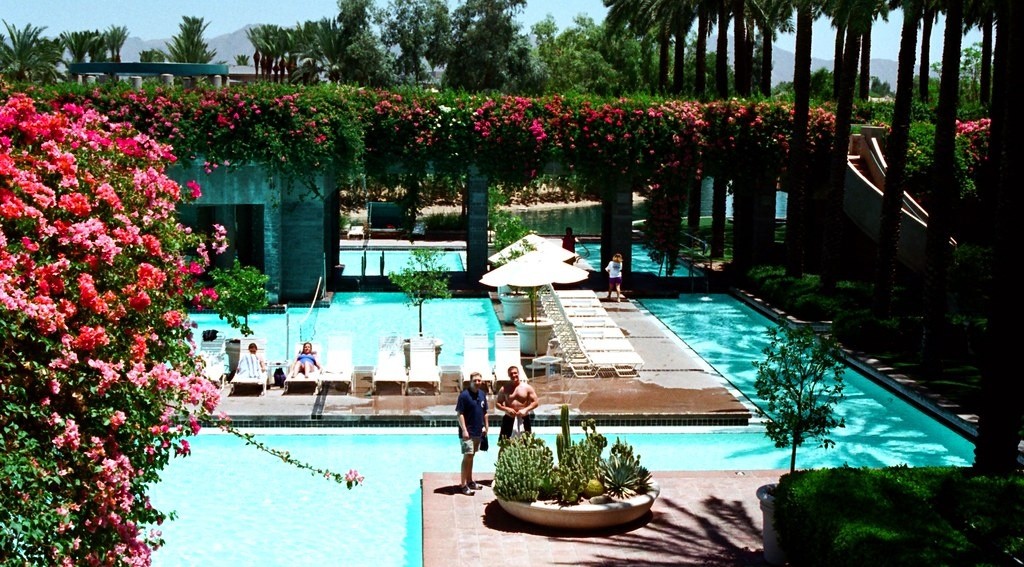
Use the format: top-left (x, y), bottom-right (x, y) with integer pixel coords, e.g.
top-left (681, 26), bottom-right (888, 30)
top-left (605, 254), bottom-right (623, 303)
top-left (496, 366), bottom-right (539, 461)
top-left (455, 372), bottom-right (489, 496)
top-left (237, 342), bottom-right (265, 378)
top-left (562, 227), bottom-right (576, 265)
top-left (291, 341), bottom-right (321, 379)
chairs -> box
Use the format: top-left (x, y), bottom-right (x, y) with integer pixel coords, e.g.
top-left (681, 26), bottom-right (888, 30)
top-left (346, 225), bottom-right (365, 240)
top-left (457, 330), bottom-right (530, 393)
top-left (280, 330), bottom-right (356, 395)
top-left (368, 330), bottom-right (442, 396)
top-left (414, 222), bottom-right (427, 239)
top-left (198, 329), bottom-right (267, 397)
top-left (540, 284), bottom-right (645, 379)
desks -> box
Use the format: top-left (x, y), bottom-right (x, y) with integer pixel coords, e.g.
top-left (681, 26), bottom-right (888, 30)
top-left (532, 356), bottom-right (563, 383)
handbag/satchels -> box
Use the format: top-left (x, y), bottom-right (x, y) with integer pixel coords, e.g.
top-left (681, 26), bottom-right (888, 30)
top-left (480, 432), bottom-right (488, 451)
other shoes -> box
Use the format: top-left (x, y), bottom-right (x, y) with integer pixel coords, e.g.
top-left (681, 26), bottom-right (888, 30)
top-left (466, 481), bottom-right (482, 490)
top-left (459, 484), bottom-right (475, 495)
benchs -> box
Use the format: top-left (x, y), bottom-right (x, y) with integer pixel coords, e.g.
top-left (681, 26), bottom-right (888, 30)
top-left (363, 220), bottom-right (407, 239)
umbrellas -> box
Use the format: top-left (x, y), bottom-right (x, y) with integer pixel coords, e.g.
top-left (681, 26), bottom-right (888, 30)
top-left (479, 232), bottom-right (590, 357)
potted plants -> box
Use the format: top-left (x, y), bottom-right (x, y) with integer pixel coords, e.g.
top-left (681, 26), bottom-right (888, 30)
top-left (491, 404), bottom-right (660, 530)
top-left (486, 185), bottom-right (511, 257)
top-left (494, 215), bottom-right (554, 356)
top-left (200, 254), bottom-right (274, 376)
top-left (388, 245), bottom-right (454, 377)
top-left (751, 309), bottom-right (849, 565)
